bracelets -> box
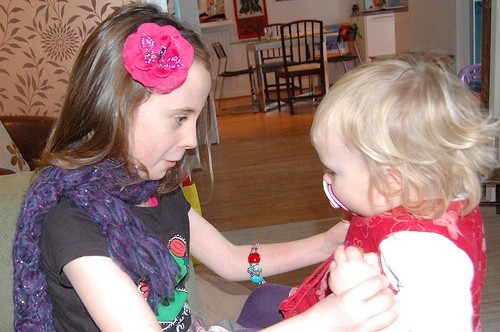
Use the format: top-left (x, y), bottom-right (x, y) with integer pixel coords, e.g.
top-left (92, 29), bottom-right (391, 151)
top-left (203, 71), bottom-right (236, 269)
top-left (247, 242), bottom-right (267, 286)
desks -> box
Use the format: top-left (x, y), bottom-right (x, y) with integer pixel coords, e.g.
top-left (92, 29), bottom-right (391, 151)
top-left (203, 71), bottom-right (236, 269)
top-left (253, 33), bottom-right (346, 110)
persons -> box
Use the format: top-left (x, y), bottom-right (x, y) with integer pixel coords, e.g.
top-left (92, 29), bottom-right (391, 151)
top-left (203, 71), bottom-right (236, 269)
top-left (210, 40), bottom-right (500, 332)
top-left (11, 4), bottom-right (398, 332)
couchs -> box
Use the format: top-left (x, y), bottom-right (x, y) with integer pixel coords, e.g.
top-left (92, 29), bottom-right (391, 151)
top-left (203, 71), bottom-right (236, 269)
top-left (0, 172), bottom-right (207, 332)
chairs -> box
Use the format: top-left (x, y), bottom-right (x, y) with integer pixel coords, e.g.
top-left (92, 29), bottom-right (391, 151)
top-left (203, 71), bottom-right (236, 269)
top-left (212, 42), bottom-right (255, 112)
top-left (258, 23), bottom-right (296, 101)
top-left (275, 19), bottom-right (325, 115)
top-left (315, 25), bottom-right (362, 72)
